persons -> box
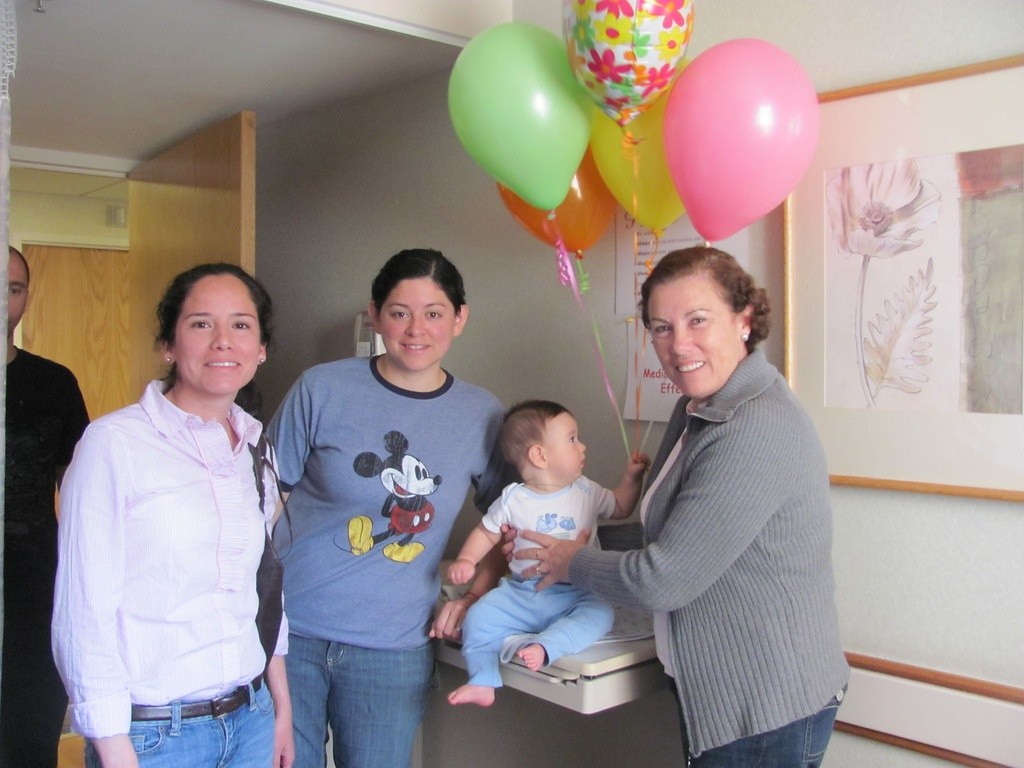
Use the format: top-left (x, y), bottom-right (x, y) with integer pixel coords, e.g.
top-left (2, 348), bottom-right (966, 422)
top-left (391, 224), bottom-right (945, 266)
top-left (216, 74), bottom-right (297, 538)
top-left (444, 399), bottom-right (655, 708)
top-left (499, 244), bottom-right (852, 768)
top-left (264, 244), bottom-right (526, 768)
top-left (48, 265), bottom-right (298, 768)
top-left (1, 243), bottom-right (90, 768)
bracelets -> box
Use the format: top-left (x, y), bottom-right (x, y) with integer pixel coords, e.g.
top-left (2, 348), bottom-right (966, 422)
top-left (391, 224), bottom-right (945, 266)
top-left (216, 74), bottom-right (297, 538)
top-left (463, 592), bottom-right (479, 602)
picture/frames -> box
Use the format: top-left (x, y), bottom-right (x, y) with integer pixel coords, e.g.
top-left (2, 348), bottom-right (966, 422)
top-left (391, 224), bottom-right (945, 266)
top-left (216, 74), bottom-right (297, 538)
top-left (778, 51), bottom-right (1023, 506)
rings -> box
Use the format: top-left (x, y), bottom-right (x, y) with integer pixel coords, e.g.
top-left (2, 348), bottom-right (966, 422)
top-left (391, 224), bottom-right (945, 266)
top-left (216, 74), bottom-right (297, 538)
top-left (455, 625), bottom-right (462, 632)
top-left (535, 548), bottom-right (542, 560)
top-left (537, 563), bottom-right (544, 574)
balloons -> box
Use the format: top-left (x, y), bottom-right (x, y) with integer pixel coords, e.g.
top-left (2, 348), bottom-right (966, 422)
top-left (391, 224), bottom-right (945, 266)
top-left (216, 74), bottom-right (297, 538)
top-left (495, 141), bottom-right (624, 258)
top-left (663, 37), bottom-right (821, 245)
top-left (559, 0), bottom-right (697, 141)
top-left (446, 21), bottom-right (597, 213)
top-left (588, 51), bottom-right (701, 239)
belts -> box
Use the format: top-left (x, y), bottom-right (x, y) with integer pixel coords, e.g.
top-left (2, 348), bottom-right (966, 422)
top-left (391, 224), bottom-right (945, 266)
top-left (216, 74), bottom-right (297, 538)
top-left (120, 675), bottom-right (269, 720)
